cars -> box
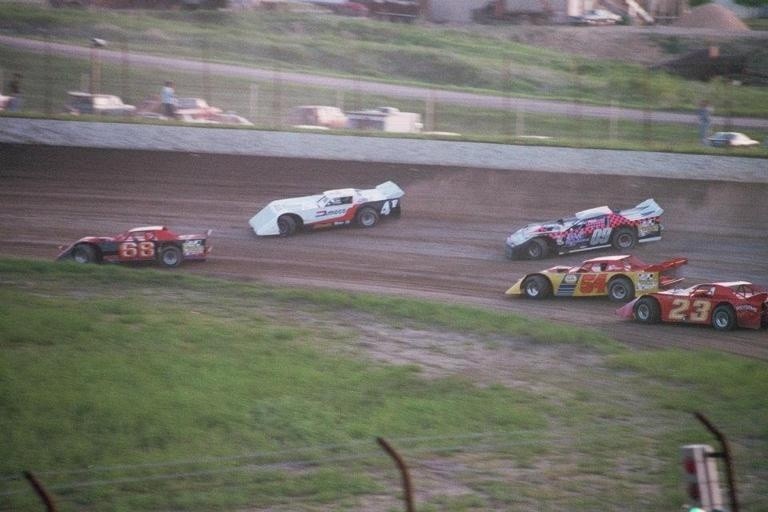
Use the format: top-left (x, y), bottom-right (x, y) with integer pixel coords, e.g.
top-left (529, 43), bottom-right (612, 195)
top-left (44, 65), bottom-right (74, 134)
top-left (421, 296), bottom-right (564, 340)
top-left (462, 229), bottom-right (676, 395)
top-left (708, 127), bottom-right (762, 148)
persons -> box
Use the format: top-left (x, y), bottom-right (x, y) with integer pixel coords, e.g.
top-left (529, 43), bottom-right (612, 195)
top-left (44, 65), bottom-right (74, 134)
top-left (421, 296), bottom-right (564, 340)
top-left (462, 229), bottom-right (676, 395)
top-left (9, 72), bottom-right (24, 113)
top-left (330, 196), bottom-right (352, 205)
top-left (697, 99), bottom-right (713, 145)
top-left (158, 79), bottom-right (177, 118)
top-left (132, 231), bottom-right (154, 242)
top-left (587, 261), bottom-right (607, 273)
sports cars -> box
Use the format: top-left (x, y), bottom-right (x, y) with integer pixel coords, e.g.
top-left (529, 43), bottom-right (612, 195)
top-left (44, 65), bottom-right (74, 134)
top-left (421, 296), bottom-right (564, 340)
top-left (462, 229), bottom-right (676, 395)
top-left (498, 252), bottom-right (695, 302)
top-left (612, 279), bottom-right (768, 333)
top-left (52, 222), bottom-right (211, 271)
top-left (247, 179), bottom-right (405, 240)
top-left (503, 194), bottom-right (665, 263)
